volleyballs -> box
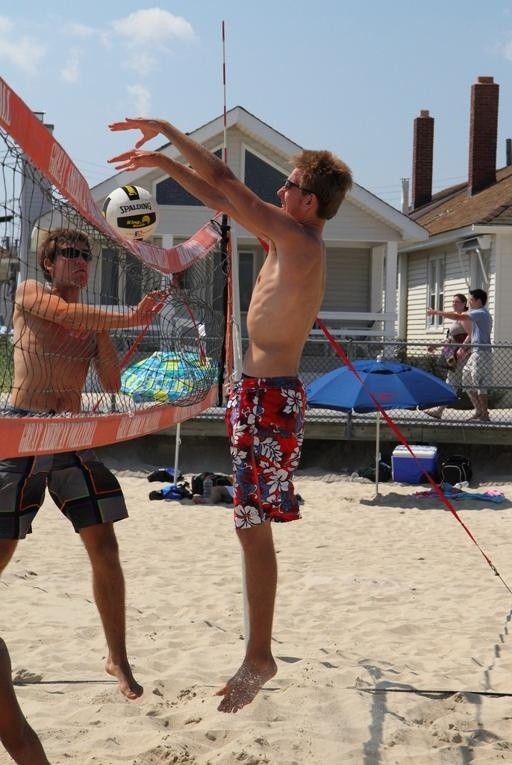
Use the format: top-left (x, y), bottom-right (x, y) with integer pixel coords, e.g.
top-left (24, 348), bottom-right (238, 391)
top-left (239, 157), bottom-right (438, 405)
top-left (101, 186), bottom-right (159, 241)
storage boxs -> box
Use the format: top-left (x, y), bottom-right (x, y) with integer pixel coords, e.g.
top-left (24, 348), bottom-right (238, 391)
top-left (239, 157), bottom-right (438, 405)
top-left (391, 444), bottom-right (439, 484)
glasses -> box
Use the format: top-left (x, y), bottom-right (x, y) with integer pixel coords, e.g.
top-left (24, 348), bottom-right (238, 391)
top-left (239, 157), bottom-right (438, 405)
top-left (56, 248), bottom-right (93, 260)
top-left (284, 180), bottom-right (320, 199)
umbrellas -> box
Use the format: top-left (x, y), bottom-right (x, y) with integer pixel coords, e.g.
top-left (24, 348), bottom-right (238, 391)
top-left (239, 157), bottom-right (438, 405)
top-left (301, 353), bottom-right (462, 501)
top-left (360, 490), bottom-right (512, 513)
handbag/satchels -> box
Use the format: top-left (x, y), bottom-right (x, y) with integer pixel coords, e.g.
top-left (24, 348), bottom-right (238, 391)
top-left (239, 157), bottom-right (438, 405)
top-left (192, 472), bottom-right (230, 494)
top-left (364, 462), bottom-right (391, 481)
top-left (441, 455), bottom-right (472, 483)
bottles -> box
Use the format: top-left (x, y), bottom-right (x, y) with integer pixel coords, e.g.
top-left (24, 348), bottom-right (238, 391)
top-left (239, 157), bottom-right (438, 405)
top-left (203, 476), bottom-right (213, 503)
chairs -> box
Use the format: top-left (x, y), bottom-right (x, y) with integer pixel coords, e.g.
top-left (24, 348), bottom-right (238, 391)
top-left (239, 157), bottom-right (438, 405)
top-left (303, 308), bottom-right (384, 357)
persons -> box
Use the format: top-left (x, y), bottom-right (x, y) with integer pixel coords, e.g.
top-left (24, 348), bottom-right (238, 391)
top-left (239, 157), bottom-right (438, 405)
top-left (426, 288), bottom-right (494, 423)
top-left (105, 115), bottom-right (352, 715)
top-left (423, 294), bottom-right (485, 420)
top-left (1, 227), bottom-right (168, 699)
top-left (0, 637), bottom-right (52, 765)
